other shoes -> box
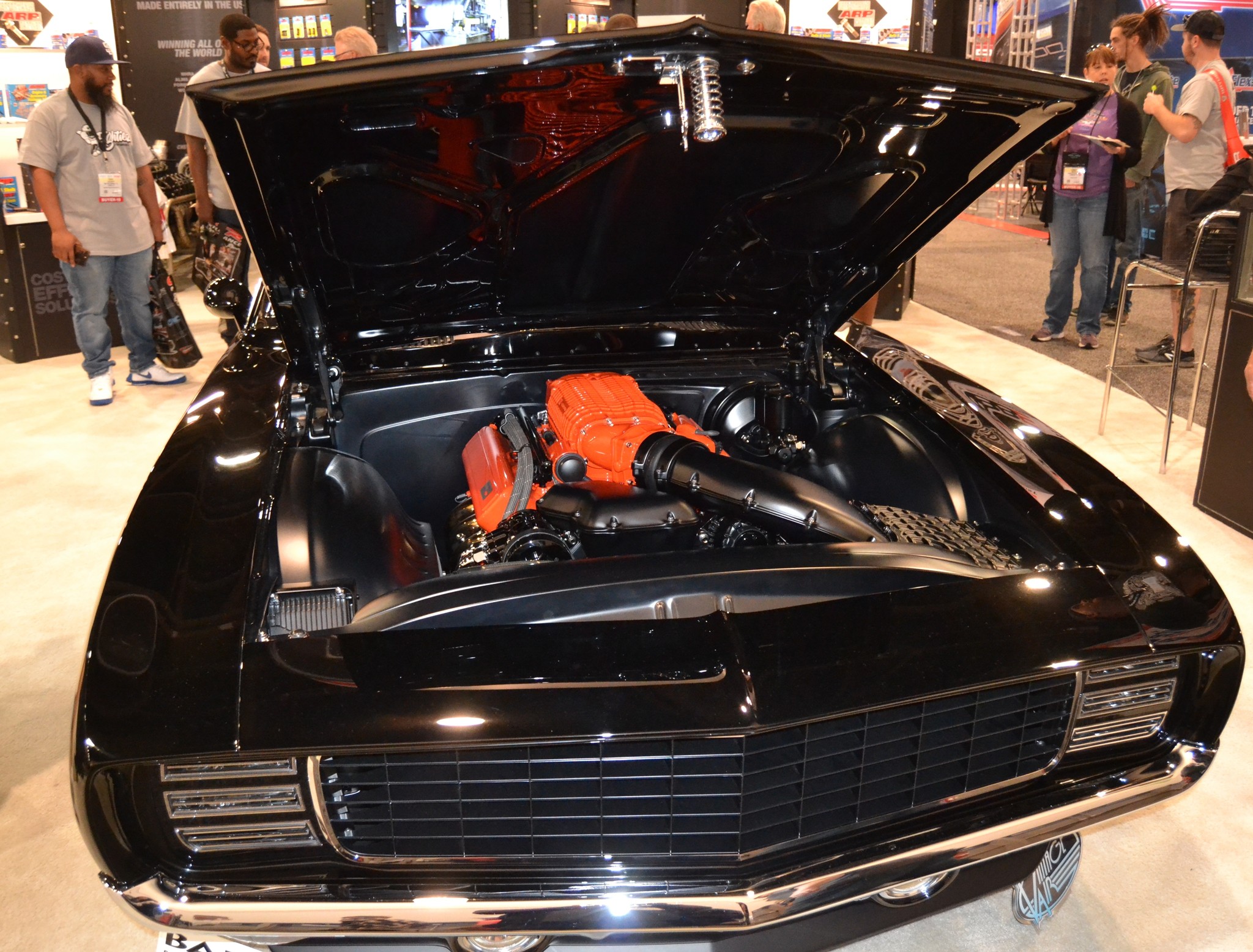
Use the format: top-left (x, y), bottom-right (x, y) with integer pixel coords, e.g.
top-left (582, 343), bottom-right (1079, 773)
top-left (217, 317), bottom-right (234, 342)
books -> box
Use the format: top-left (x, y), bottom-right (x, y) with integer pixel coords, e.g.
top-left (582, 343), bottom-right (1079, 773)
top-left (0, 27), bottom-right (99, 212)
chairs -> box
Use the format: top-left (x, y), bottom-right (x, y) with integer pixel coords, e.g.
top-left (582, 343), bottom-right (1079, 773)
top-left (1096, 208), bottom-right (1240, 477)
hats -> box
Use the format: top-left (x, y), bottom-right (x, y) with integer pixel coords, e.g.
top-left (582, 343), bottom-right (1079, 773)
top-left (1184, 10), bottom-right (1227, 43)
top-left (65, 33), bottom-right (132, 74)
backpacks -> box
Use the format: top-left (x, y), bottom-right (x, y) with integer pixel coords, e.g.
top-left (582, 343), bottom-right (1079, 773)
top-left (1176, 158), bottom-right (1253, 282)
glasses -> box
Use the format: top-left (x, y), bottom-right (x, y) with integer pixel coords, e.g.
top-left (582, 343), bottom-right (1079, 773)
top-left (1086, 43), bottom-right (1117, 58)
top-left (333, 46), bottom-right (356, 62)
top-left (227, 37), bottom-right (265, 52)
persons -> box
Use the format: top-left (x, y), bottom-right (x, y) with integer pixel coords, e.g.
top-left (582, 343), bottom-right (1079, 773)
top-left (1040, 42), bottom-right (1145, 350)
top-left (1134, 9), bottom-right (1236, 369)
top-left (15, 34), bottom-right (186, 406)
top-left (581, 22), bottom-right (605, 31)
top-left (333, 25), bottom-right (379, 61)
top-left (1069, 2), bottom-right (1200, 326)
top-left (744, 0), bottom-right (787, 34)
top-left (605, 14), bottom-right (638, 30)
top-left (255, 24), bottom-right (271, 67)
top-left (174, 12), bottom-right (272, 349)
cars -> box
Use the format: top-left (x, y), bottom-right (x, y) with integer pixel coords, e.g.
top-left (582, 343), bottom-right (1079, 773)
top-left (70, 13), bottom-right (1249, 936)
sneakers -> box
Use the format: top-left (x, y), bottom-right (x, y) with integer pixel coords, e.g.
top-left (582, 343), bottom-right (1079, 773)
top-left (88, 366), bottom-right (117, 406)
top-left (1135, 333), bottom-right (1177, 355)
top-left (127, 365), bottom-right (187, 386)
top-left (1105, 307), bottom-right (1130, 326)
top-left (1077, 329), bottom-right (1101, 350)
top-left (1030, 323), bottom-right (1067, 343)
top-left (1135, 344), bottom-right (1196, 371)
top-left (1070, 303), bottom-right (1081, 315)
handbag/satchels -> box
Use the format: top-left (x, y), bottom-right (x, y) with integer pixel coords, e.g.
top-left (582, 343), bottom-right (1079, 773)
top-left (190, 210), bottom-right (246, 312)
top-left (145, 240), bottom-right (203, 369)
